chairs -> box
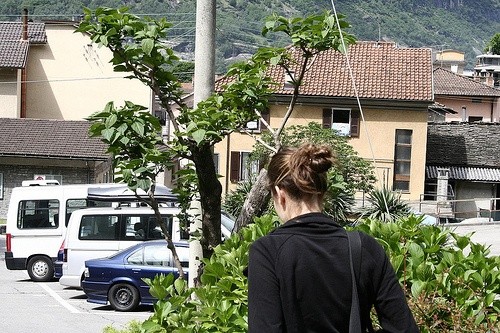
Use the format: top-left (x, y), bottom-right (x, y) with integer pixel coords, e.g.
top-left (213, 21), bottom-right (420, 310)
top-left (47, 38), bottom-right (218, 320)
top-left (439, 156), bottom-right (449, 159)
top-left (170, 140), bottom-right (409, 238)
top-left (22, 199), bottom-right (191, 266)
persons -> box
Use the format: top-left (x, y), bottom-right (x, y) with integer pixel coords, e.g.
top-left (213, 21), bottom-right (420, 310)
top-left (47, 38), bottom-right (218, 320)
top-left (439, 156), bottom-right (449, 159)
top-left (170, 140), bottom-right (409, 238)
top-left (248, 141), bottom-right (422, 333)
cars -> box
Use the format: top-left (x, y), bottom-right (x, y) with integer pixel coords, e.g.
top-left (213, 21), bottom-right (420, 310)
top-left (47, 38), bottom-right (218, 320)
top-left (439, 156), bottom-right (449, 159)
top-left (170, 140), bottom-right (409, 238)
top-left (80, 240), bottom-right (189, 311)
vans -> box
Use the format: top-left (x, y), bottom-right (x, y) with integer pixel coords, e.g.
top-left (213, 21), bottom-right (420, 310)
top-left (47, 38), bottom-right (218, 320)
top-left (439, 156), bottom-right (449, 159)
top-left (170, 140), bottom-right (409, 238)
top-left (5, 180), bottom-right (236, 282)
top-left (54, 207), bottom-right (231, 291)
